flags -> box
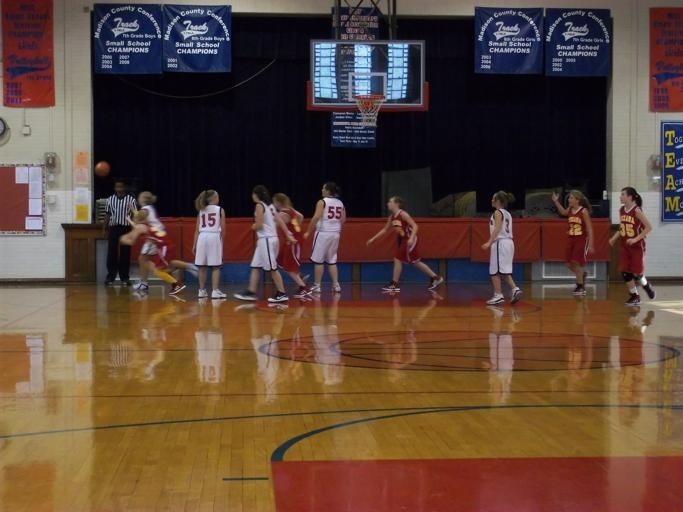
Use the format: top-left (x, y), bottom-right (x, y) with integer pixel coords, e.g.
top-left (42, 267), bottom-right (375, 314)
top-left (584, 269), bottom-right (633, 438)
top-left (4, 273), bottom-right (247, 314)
top-left (162, 6), bottom-right (232, 74)
top-left (91, 2), bottom-right (162, 75)
top-left (331, 5), bottom-right (379, 150)
top-left (472, 6), bottom-right (543, 76)
top-left (545, 8), bottom-right (613, 76)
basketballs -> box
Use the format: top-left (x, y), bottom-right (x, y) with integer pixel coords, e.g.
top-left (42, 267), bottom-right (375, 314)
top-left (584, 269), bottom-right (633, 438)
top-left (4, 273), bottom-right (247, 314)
top-left (94, 161), bottom-right (111, 177)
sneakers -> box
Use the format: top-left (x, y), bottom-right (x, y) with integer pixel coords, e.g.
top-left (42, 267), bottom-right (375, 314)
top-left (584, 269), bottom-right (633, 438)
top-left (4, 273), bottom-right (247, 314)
top-left (511, 287), bottom-right (522, 304)
top-left (428, 275), bottom-right (444, 290)
top-left (625, 293), bottom-right (642, 305)
top-left (382, 281), bottom-right (401, 290)
top-left (642, 282), bottom-right (656, 299)
top-left (486, 293), bottom-right (504, 305)
top-left (573, 283), bottom-right (587, 295)
top-left (105, 264), bottom-right (340, 302)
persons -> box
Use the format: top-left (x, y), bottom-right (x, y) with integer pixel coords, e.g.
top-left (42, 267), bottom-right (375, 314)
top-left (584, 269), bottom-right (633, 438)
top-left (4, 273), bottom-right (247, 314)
top-left (186, 185), bottom-right (226, 303)
top-left (232, 182), bottom-right (347, 304)
top-left (474, 190), bottom-right (523, 308)
top-left (606, 187), bottom-right (655, 306)
top-left (119, 212), bottom-right (184, 298)
top-left (364, 195), bottom-right (445, 296)
top-left (549, 188), bottom-right (593, 298)
top-left (99, 181), bottom-right (137, 286)
top-left (122, 191), bottom-right (199, 292)
